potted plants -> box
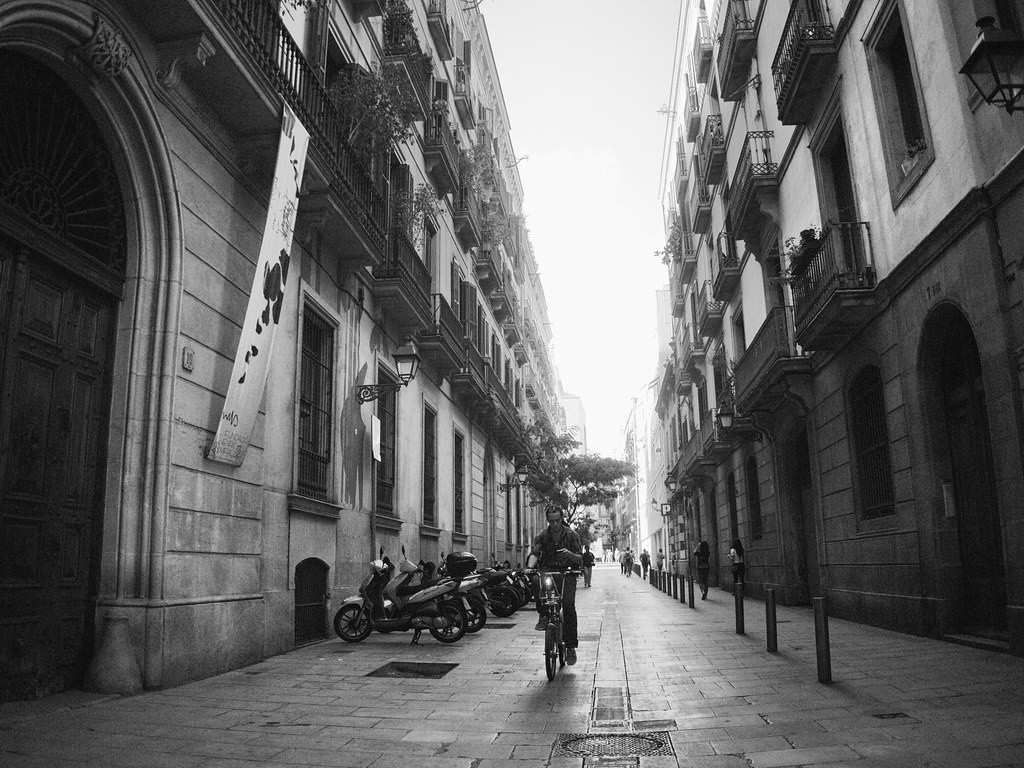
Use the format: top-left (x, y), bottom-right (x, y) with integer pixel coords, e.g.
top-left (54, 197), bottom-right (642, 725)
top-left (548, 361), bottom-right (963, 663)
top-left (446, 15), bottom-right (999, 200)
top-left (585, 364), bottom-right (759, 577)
top-left (781, 227), bottom-right (821, 274)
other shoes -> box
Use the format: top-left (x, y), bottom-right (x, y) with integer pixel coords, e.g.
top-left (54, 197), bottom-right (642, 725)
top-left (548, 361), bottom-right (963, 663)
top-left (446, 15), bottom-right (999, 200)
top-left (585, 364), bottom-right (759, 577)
top-left (702, 593), bottom-right (706, 600)
top-left (567, 650), bottom-right (576, 665)
top-left (535, 617), bottom-right (547, 629)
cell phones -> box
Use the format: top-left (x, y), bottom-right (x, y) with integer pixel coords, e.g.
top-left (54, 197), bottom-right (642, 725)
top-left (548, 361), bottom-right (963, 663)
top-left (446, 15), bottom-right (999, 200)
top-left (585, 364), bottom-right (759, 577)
top-left (556, 550), bottom-right (563, 553)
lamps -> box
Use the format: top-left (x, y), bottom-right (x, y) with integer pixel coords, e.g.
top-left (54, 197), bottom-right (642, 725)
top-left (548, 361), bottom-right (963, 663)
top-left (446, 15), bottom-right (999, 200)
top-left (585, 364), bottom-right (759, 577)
top-left (665, 472), bottom-right (694, 499)
top-left (356, 341), bottom-right (422, 405)
top-left (651, 498), bottom-right (661, 511)
top-left (499, 467), bottom-right (530, 493)
top-left (715, 400), bottom-right (763, 443)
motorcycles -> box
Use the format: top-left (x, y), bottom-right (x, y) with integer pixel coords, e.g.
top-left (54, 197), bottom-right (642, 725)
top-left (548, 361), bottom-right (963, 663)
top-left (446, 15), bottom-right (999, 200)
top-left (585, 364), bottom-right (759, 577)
top-left (333, 544), bottom-right (537, 645)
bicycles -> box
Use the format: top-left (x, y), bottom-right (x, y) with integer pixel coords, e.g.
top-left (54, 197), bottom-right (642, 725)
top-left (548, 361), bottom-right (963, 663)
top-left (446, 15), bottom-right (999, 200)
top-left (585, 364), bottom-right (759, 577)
top-left (522, 569), bottom-right (581, 681)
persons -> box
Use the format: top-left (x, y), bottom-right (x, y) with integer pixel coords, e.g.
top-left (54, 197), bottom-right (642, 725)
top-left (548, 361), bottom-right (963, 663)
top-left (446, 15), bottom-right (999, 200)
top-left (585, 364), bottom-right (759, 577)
top-left (519, 504), bottom-right (585, 667)
top-left (580, 545), bottom-right (595, 588)
top-left (601, 546), bottom-right (677, 580)
top-left (693, 541), bottom-right (711, 600)
top-left (727, 539), bottom-right (746, 598)
